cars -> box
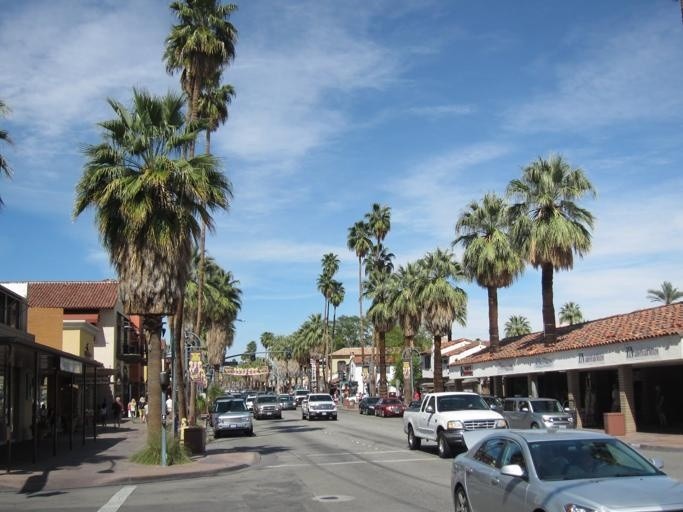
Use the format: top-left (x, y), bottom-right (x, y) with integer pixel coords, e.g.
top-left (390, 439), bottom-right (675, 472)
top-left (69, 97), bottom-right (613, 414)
top-left (374, 399), bottom-right (405, 418)
top-left (450, 428), bottom-right (681, 512)
top-left (212, 400), bottom-right (253, 437)
top-left (225, 389), bottom-right (311, 410)
top-left (254, 398), bottom-right (280, 421)
top-left (359, 398), bottom-right (378, 416)
top-left (302, 393), bottom-right (337, 421)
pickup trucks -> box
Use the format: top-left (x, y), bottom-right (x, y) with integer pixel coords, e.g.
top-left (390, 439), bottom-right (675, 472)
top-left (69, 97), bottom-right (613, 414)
top-left (402, 392), bottom-right (505, 458)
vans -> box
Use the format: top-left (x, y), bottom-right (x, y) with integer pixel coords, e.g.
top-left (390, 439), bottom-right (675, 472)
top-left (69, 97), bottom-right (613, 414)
top-left (494, 397), bottom-right (573, 429)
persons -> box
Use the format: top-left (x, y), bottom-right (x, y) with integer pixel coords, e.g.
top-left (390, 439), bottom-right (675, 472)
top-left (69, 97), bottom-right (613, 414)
top-left (610, 383), bottom-right (620, 412)
top-left (330, 387), bottom-right (367, 409)
top-left (38, 403), bottom-right (48, 438)
top-left (653, 384), bottom-right (669, 429)
top-left (97, 393), bottom-right (174, 430)
